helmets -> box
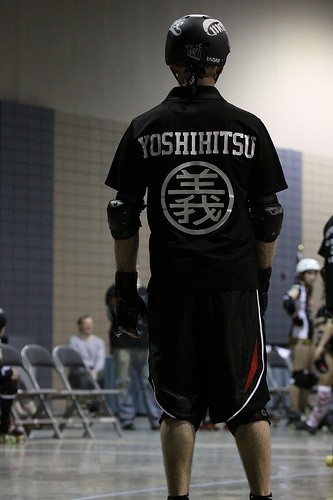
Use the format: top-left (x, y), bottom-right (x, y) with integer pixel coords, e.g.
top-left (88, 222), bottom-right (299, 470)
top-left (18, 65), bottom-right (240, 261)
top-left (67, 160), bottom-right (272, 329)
top-left (295, 258), bottom-right (322, 278)
top-left (165, 14), bottom-right (230, 65)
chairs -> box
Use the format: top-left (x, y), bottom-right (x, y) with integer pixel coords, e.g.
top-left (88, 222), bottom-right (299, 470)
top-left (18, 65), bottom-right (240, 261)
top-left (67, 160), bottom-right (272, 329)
top-left (265, 358), bottom-right (294, 426)
top-left (0, 341), bottom-right (125, 437)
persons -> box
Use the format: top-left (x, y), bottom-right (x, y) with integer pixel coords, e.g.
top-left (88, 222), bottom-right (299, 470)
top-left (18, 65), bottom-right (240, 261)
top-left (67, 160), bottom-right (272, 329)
top-left (104, 284), bottom-right (162, 432)
top-left (304, 216), bottom-right (333, 436)
top-left (67, 316), bottom-right (107, 420)
top-left (283, 258), bottom-right (322, 416)
top-left (104, 12), bottom-right (283, 500)
top-left (0, 309), bottom-right (25, 435)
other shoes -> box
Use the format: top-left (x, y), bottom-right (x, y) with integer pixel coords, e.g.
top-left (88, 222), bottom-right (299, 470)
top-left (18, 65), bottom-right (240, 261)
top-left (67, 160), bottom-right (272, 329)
top-left (287, 410), bottom-right (333, 435)
top-left (150, 417), bottom-right (161, 430)
top-left (120, 418), bottom-right (135, 431)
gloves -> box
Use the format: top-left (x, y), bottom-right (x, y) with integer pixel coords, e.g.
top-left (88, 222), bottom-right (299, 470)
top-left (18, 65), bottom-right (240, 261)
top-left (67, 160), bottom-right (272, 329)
top-left (294, 315), bottom-right (304, 328)
top-left (114, 271), bottom-right (148, 339)
